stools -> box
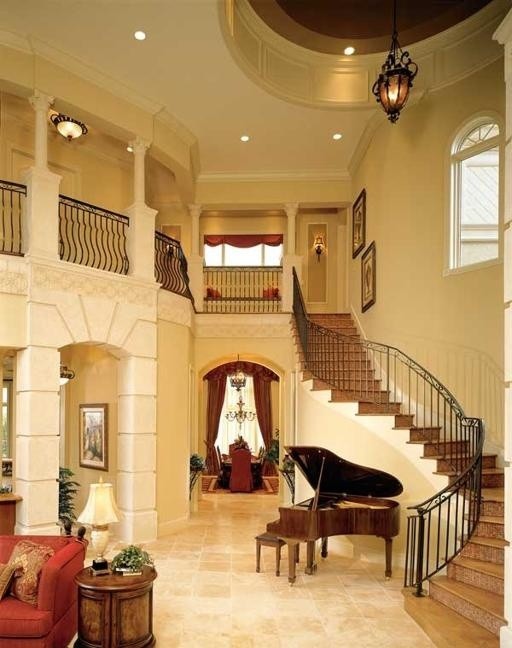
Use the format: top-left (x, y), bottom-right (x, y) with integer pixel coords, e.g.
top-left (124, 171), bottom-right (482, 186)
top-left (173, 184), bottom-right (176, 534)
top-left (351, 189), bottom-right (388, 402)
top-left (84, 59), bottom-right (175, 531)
top-left (254, 532), bottom-right (300, 577)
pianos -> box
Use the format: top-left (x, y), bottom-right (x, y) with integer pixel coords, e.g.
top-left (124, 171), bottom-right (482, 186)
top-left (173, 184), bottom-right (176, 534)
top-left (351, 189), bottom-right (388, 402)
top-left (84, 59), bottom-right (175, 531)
top-left (267, 446), bottom-right (403, 586)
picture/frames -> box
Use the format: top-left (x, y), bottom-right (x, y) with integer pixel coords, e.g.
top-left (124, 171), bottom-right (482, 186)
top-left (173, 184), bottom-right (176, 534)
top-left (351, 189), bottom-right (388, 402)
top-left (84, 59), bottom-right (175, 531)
top-left (78, 403), bottom-right (110, 472)
top-left (351, 187), bottom-right (378, 314)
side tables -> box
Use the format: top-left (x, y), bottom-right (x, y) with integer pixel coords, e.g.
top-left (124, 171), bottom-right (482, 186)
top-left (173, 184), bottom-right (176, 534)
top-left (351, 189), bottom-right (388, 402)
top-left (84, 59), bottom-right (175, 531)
top-left (72, 563), bottom-right (158, 648)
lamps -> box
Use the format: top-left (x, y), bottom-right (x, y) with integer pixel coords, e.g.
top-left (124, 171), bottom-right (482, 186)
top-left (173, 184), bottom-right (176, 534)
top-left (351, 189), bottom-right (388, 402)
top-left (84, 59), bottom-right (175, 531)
top-left (76, 475), bottom-right (123, 576)
top-left (229, 354), bottom-right (247, 392)
top-left (371, 0), bottom-right (419, 125)
top-left (313, 234), bottom-right (326, 262)
top-left (50, 112), bottom-right (89, 142)
top-left (225, 394), bottom-right (257, 430)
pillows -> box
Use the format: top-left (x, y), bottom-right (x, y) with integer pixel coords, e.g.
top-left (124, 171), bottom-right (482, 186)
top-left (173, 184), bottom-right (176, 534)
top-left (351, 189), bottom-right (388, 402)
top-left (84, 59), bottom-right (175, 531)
top-left (0, 540), bottom-right (56, 609)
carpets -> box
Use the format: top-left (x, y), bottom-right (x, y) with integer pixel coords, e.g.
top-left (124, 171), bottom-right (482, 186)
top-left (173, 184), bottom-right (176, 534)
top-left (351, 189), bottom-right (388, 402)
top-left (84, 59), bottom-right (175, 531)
top-left (201, 477), bottom-right (280, 496)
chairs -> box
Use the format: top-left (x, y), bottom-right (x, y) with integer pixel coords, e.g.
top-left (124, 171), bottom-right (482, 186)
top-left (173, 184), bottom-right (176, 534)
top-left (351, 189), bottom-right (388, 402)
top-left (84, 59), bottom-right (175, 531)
top-left (216, 444), bottom-right (270, 492)
top-left (0, 533), bottom-right (89, 648)
